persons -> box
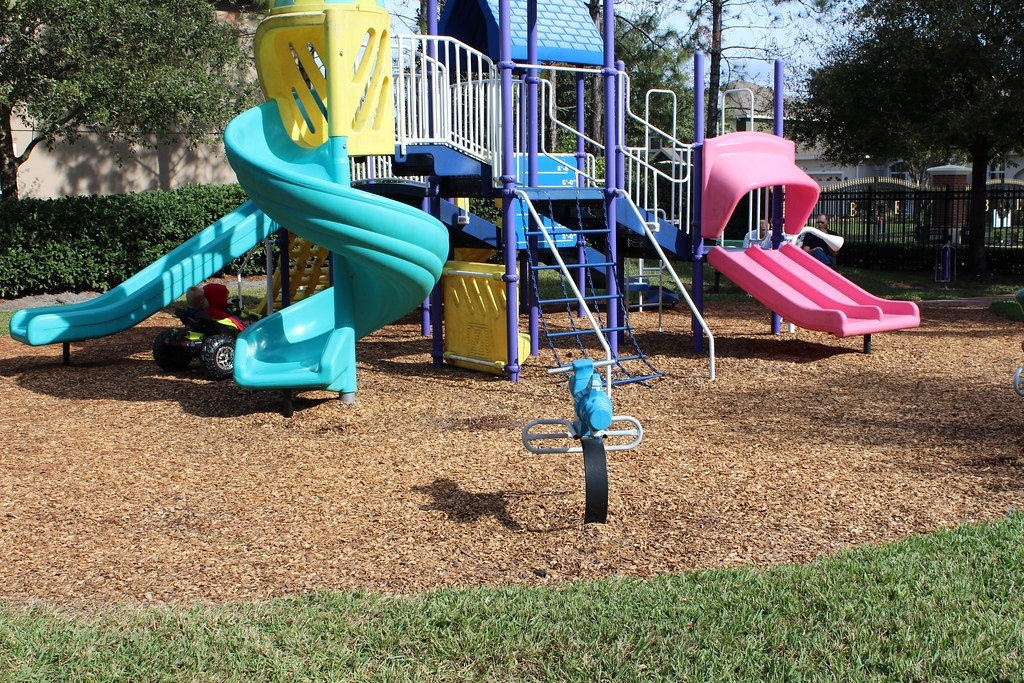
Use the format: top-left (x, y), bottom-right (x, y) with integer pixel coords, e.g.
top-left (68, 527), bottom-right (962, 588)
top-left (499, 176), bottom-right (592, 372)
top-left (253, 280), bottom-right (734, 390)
top-left (202, 283), bottom-right (250, 331)
top-left (803, 214), bottom-right (839, 270)
top-left (742, 220), bottom-right (773, 249)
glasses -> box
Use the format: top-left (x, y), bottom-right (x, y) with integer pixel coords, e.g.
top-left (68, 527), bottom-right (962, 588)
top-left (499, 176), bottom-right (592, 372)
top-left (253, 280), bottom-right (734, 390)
top-left (817, 221), bottom-right (828, 224)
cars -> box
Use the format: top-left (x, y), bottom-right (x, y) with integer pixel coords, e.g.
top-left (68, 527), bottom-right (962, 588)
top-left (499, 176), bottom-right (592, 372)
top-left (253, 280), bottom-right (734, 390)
top-left (155, 285), bottom-right (271, 380)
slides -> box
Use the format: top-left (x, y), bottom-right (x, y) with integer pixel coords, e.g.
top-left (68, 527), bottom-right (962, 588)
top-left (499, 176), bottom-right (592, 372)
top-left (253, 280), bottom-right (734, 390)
top-left (707, 243), bottom-right (920, 337)
top-left (224, 87), bottom-right (450, 396)
top-left (7, 198), bottom-right (281, 347)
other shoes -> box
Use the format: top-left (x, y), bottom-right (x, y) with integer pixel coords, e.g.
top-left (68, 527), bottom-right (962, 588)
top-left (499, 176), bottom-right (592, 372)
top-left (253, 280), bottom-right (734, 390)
top-left (745, 291), bottom-right (752, 298)
